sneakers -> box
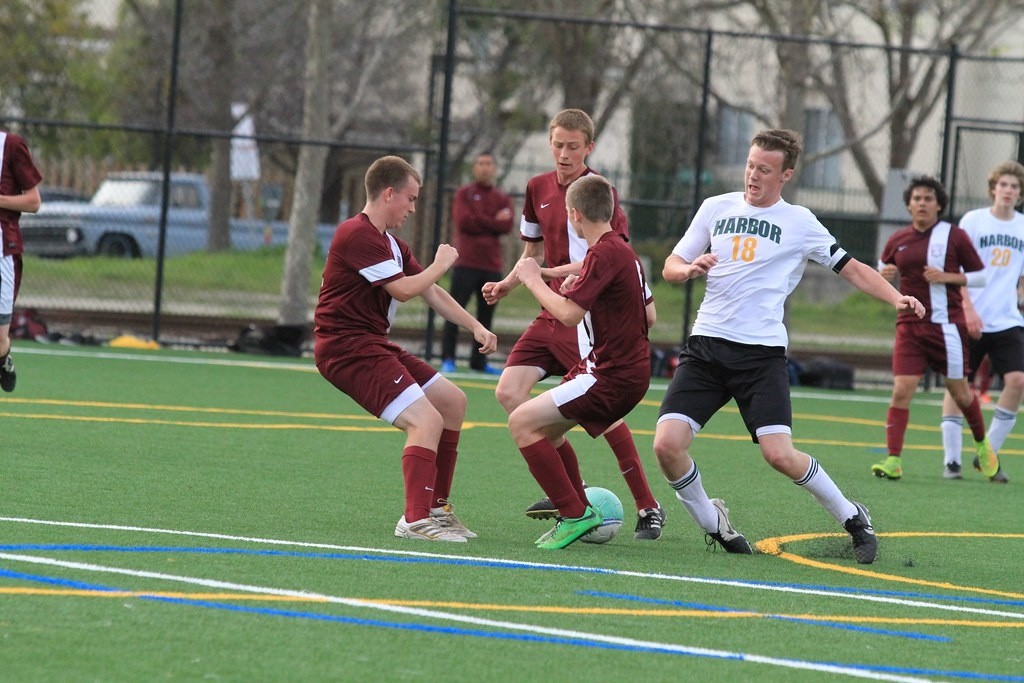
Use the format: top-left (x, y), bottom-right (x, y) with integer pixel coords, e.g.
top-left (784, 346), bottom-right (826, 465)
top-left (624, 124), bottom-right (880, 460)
top-left (871, 458), bottom-right (903, 480)
top-left (944, 463), bottom-right (963, 480)
top-left (632, 500), bottom-right (668, 542)
top-left (972, 456), bottom-right (1009, 483)
top-left (533, 505), bottom-right (604, 550)
top-left (841, 501), bottom-right (877, 566)
top-left (394, 515), bottom-right (469, 543)
top-left (974, 439), bottom-right (1000, 477)
top-left (525, 497), bottom-right (560, 520)
top-left (705, 498), bottom-right (753, 555)
top-left (429, 498), bottom-right (479, 538)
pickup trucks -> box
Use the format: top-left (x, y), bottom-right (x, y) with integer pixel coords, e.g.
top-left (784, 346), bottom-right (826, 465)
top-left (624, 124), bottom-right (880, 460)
top-left (18, 166), bottom-right (336, 261)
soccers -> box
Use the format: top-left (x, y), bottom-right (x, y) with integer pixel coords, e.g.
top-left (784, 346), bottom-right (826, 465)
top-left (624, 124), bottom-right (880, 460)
top-left (580, 486), bottom-right (625, 544)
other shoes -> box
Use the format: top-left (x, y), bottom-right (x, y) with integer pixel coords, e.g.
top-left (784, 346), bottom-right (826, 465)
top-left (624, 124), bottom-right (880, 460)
top-left (0, 354), bottom-right (16, 393)
top-left (439, 359), bottom-right (458, 373)
top-left (474, 365), bottom-right (504, 376)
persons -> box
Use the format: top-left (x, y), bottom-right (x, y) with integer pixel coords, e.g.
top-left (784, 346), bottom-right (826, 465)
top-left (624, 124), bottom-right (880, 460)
top-left (654, 129), bottom-right (926, 564)
top-left (870, 177), bottom-right (1000, 480)
top-left (507, 174), bottom-right (656, 548)
top-left (442, 150), bottom-right (514, 376)
top-left (313, 156), bottom-right (498, 543)
top-left (0, 131), bottom-right (43, 393)
top-left (482, 110), bottom-right (667, 543)
top-left (941, 160), bottom-right (1024, 482)
